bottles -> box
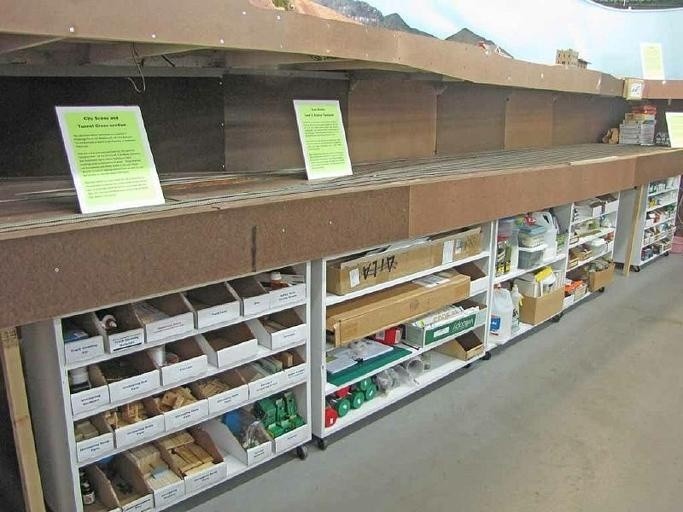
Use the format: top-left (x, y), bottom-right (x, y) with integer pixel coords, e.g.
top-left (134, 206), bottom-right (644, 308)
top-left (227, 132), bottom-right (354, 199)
top-left (510, 284), bottom-right (519, 332)
top-left (79, 472), bottom-right (96, 505)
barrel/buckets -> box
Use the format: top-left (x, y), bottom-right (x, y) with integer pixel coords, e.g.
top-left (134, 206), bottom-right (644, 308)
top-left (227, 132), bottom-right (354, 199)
top-left (531, 210), bottom-right (557, 262)
top-left (489, 285), bottom-right (512, 342)
